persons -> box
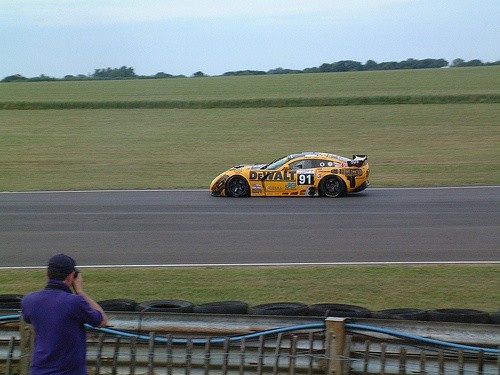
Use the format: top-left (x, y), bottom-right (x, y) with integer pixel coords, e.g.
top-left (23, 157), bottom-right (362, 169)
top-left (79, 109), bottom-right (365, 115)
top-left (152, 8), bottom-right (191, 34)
top-left (298, 159), bottom-right (311, 170)
top-left (20, 254), bottom-right (108, 375)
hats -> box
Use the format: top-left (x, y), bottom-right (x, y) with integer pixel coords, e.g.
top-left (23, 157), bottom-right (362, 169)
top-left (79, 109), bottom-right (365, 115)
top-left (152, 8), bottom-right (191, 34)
top-left (46, 253), bottom-right (75, 275)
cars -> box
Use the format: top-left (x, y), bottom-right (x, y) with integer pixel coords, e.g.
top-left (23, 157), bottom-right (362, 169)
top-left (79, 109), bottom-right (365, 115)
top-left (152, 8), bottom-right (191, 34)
top-left (208, 151), bottom-right (370, 199)
